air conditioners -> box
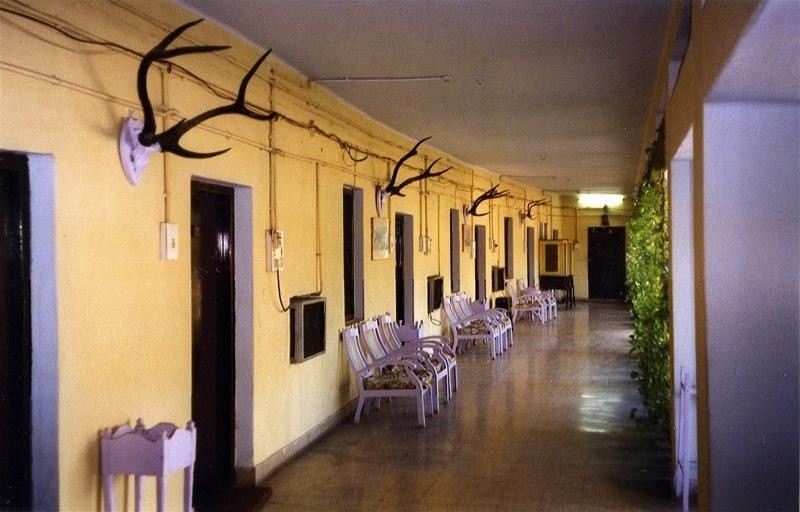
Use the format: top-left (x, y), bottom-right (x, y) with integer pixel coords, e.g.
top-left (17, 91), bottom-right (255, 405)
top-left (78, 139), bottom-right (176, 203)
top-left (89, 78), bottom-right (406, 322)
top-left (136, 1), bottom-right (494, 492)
top-left (493, 268), bottom-right (504, 291)
top-left (289, 296), bottom-right (326, 363)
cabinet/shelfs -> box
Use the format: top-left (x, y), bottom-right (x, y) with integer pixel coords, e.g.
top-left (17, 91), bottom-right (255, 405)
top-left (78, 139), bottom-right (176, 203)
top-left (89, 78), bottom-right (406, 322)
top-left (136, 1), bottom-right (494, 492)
top-left (674, 365), bottom-right (698, 512)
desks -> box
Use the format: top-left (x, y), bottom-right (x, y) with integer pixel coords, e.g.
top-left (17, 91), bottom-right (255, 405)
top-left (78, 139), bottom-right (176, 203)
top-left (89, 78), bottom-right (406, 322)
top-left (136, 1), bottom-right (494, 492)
top-left (541, 276), bottom-right (577, 310)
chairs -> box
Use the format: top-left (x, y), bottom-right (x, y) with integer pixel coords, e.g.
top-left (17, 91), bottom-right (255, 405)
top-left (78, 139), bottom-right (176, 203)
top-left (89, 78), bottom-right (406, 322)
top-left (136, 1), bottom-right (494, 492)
top-left (341, 276), bottom-right (557, 427)
